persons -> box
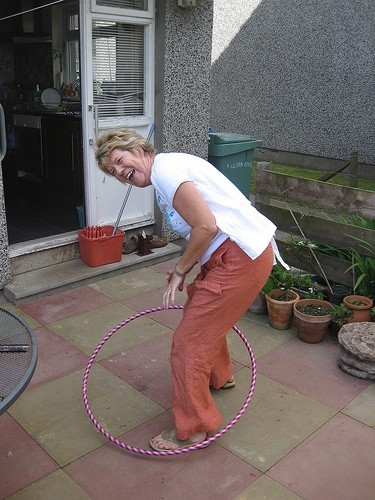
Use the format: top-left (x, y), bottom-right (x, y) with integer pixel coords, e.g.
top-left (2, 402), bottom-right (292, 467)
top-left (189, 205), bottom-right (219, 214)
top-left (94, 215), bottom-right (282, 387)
top-left (95, 127), bottom-right (277, 452)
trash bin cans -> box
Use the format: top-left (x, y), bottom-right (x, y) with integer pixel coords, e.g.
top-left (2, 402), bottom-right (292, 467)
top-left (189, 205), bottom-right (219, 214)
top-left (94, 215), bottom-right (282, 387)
top-left (207, 131), bottom-right (264, 199)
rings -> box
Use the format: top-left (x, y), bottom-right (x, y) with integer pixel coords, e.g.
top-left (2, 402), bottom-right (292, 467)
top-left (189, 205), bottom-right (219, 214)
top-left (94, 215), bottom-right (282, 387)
top-left (167, 292), bottom-right (170, 295)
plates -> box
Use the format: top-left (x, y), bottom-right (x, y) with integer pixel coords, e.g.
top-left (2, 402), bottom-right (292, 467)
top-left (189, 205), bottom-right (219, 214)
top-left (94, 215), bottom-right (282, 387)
top-left (40, 88), bottom-right (62, 109)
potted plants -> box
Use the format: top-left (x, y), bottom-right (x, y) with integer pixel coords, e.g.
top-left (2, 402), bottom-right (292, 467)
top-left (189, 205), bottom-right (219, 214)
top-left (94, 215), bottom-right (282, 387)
top-left (293, 299), bottom-right (335, 344)
top-left (344, 296), bottom-right (373, 323)
top-left (265, 288), bottom-right (299, 330)
top-left (249, 262), bottom-right (292, 315)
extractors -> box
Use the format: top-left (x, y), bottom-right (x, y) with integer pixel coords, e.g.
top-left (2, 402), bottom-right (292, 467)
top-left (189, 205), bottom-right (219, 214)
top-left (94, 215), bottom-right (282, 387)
top-left (12, 0), bottom-right (52, 45)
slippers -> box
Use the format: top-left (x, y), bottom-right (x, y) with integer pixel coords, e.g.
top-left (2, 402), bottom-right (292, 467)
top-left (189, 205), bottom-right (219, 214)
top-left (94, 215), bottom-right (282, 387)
top-left (148, 428), bottom-right (210, 452)
top-left (222, 377), bottom-right (235, 389)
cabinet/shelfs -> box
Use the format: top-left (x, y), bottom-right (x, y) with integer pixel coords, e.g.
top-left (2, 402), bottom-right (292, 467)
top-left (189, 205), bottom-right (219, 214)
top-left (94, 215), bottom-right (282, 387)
top-left (253, 160), bottom-right (375, 288)
top-left (41, 117), bottom-right (84, 192)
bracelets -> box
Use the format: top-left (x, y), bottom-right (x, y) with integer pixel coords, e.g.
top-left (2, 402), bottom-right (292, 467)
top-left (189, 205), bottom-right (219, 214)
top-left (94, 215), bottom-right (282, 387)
top-left (173, 262), bottom-right (185, 277)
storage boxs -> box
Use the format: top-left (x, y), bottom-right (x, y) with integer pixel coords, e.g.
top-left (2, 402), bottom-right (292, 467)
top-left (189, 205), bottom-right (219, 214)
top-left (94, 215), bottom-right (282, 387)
top-left (76, 225), bottom-right (124, 268)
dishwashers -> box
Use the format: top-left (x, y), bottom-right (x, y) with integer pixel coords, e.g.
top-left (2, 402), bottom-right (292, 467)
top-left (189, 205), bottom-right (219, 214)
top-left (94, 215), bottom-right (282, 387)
top-left (13, 114), bottom-right (46, 182)
